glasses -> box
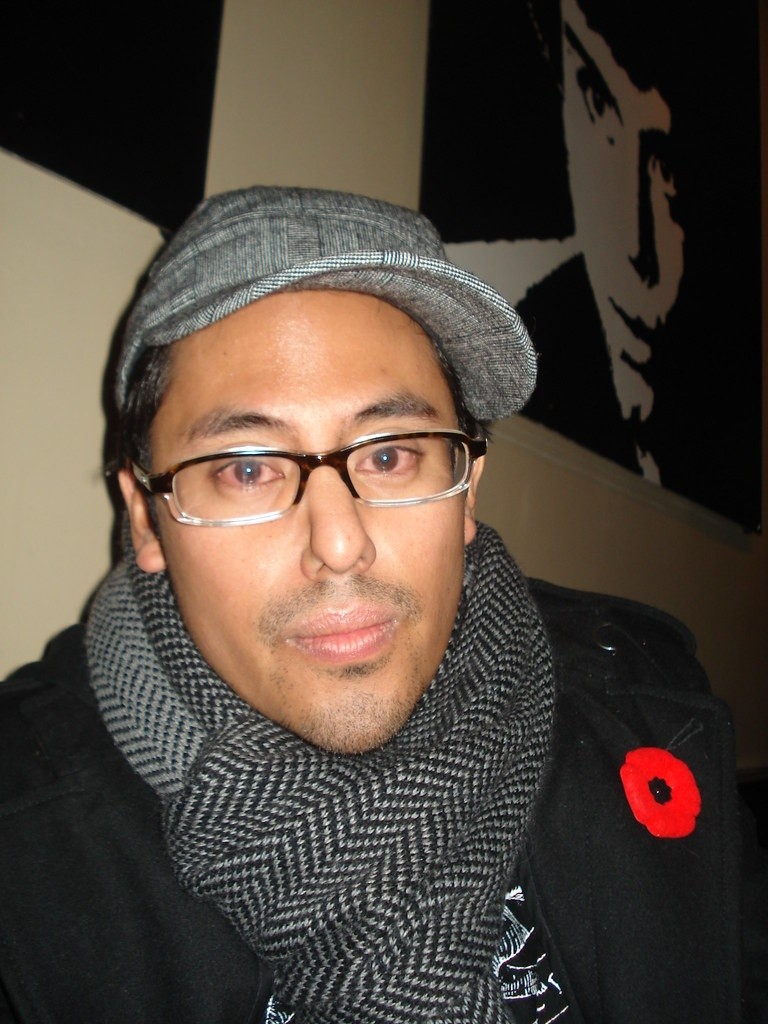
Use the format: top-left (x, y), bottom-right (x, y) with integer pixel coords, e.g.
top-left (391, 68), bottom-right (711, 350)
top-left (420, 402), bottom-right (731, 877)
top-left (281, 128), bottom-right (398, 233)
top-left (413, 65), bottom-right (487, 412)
top-left (122, 429), bottom-right (489, 526)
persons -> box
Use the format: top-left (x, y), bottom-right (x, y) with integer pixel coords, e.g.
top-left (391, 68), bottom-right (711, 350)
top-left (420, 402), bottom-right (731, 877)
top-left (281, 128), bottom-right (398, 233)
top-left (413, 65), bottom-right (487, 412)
top-left (0, 186), bottom-right (744, 1024)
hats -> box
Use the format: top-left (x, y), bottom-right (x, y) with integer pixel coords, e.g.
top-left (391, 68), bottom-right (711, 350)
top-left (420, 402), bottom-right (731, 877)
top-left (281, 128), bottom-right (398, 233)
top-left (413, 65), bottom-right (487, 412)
top-left (114, 185), bottom-right (538, 423)
